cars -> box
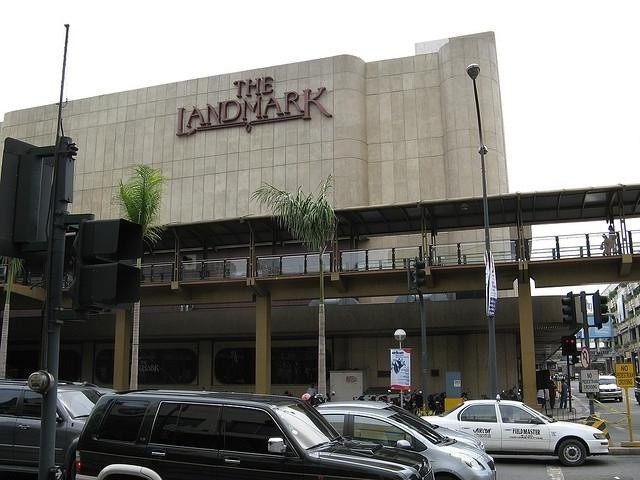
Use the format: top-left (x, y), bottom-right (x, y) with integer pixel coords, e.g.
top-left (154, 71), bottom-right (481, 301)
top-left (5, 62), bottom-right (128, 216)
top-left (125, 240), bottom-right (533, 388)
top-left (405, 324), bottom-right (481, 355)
top-left (555, 371), bottom-right (640, 405)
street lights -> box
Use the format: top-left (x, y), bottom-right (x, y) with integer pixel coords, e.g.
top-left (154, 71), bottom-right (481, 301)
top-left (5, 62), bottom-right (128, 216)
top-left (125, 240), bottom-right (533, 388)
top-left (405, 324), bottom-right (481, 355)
top-left (465, 63), bottom-right (501, 399)
top-left (393, 329), bottom-right (408, 410)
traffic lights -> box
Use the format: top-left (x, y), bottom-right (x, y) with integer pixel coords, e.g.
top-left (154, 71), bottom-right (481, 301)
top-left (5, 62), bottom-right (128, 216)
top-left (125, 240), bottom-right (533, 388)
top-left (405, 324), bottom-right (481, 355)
top-left (71, 218), bottom-right (144, 316)
top-left (419, 262), bottom-right (428, 286)
top-left (563, 336), bottom-right (576, 353)
top-left (595, 292), bottom-right (609, 324)
top-left (561, 292), bottom-right (574, 325)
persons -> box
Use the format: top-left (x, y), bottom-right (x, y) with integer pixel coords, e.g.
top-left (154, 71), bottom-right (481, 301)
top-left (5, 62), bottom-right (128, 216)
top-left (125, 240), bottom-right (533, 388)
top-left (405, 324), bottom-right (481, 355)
top-left (306, 383), bottom-right (318, 397)
top-left (601, 234), bottom-right (610, 256)
top-left (549, 381), bottom-right (557, 408)
top-left (607, 226), bottom-right (618, 255)
top-left (560, 379), bottom-right (570, 409)
